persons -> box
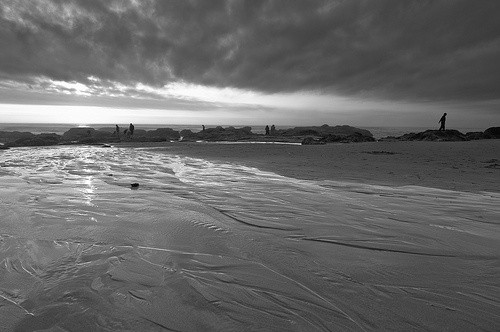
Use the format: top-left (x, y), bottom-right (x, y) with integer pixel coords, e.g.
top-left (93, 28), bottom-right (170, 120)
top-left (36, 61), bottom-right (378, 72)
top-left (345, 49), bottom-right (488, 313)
top-left (271, 125), bottom-right (275, 133)
top-left (115, 124), bottom-right (120, 133)
top-left (438, 113), bottom-right (447, 131)
top-left (265, 125), bottom-right (270, 135)
top-left (129, 123), bottom-right (134, 135)
top-left (202, 125), bottom-right (205, 132)
